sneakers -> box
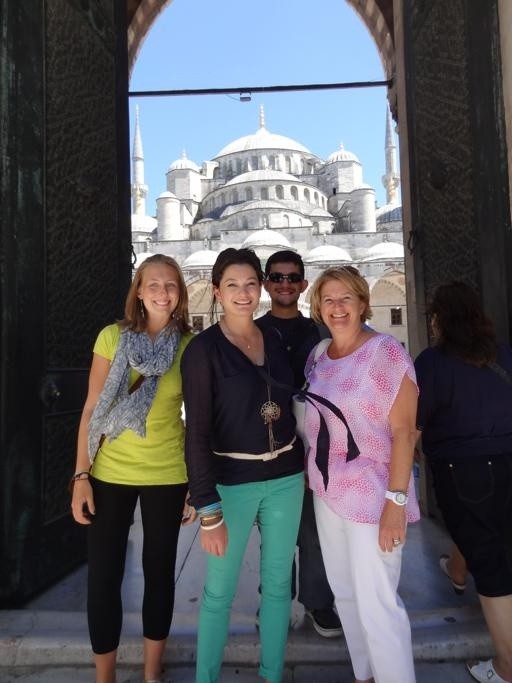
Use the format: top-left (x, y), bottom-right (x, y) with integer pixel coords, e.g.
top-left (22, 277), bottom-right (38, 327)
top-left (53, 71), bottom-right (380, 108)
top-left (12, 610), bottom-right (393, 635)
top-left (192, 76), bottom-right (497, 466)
top-left (304, 604), bottom-right (343, 638)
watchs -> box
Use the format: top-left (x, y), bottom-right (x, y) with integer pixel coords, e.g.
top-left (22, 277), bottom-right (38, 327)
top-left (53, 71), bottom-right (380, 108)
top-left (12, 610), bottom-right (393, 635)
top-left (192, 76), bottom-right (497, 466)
top-left (384, 488), bottom-right (410, 506)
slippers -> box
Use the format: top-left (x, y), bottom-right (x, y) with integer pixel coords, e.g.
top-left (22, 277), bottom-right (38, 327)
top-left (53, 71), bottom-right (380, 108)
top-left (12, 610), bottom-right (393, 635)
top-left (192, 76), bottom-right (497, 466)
top-left (466, 658), bottom-right (508, 682)
top-left (438, 553), bottom-right (467, 595)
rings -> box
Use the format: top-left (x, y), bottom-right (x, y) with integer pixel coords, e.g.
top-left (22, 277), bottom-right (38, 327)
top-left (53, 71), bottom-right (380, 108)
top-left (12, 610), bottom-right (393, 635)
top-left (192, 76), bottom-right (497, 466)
top-left (393, 538), bottom-right (401, 545)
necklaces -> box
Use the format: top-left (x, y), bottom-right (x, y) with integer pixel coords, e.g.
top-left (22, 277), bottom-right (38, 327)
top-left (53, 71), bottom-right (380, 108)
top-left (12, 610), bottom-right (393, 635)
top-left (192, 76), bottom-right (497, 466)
top-left (244, 343), bottom-right (254, 349)
top-left (221, 317), bottom-right (281, 456)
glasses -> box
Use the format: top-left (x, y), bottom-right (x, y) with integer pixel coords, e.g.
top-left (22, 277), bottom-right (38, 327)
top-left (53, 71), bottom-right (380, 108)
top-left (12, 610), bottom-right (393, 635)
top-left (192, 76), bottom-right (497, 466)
top-left (266, 272), bottom-right (304, 283)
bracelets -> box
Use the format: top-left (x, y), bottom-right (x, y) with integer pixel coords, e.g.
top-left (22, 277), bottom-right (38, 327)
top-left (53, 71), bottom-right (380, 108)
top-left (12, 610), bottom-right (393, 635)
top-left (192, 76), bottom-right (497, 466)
top-left (201, 513), bottom-right (222, 520)
top-left (73, 469), bottom-right (90, 482)
top-left (200, 517), bottom-right (225, 531)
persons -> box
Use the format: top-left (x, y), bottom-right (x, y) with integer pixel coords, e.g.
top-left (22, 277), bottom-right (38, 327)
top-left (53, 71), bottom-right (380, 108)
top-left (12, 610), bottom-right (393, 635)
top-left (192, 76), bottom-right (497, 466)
top-left (69, 252), bottom-right (197, 682)
top-left (299, 265), bottom-right (423, 681)
top-left (178, 245), bottom-right (308, 681)
top-left (413, 279), bottom-right (512, 682)
top-left (251, 248), bottom-right (344, 638)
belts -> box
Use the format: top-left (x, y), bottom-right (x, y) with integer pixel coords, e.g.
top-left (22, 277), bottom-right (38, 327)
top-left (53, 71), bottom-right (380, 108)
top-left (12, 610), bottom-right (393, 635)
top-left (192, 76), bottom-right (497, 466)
top-left (212, 436), bottom-right (297, 462)
